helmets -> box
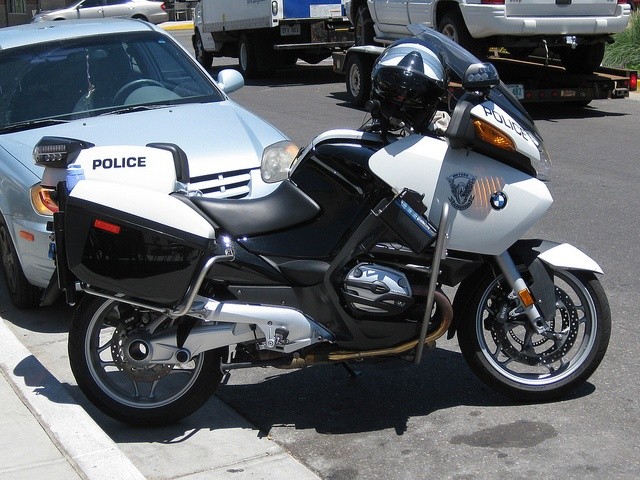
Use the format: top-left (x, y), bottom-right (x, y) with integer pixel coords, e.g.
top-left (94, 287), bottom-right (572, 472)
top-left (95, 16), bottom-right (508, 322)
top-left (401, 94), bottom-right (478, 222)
top-left (368, 36), bottom-right (447, 134)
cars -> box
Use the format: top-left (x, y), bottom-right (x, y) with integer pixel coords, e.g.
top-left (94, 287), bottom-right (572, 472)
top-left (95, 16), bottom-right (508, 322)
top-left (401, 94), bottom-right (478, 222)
top-left (30, 1), bottom-right (169, 25)
top-left (367, 0), bottom-right (630, 72)
top-left (0, 17), bottom-right (302, 310)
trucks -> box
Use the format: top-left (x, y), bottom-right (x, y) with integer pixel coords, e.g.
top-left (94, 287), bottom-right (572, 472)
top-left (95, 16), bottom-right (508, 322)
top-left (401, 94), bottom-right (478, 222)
top-left (191, 0), bottom-right (638, 104)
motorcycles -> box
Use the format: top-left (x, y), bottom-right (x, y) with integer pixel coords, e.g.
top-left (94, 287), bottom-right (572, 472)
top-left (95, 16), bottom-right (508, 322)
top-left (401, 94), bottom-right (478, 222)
top-left (54, 24), bottom-right (612, 429)
